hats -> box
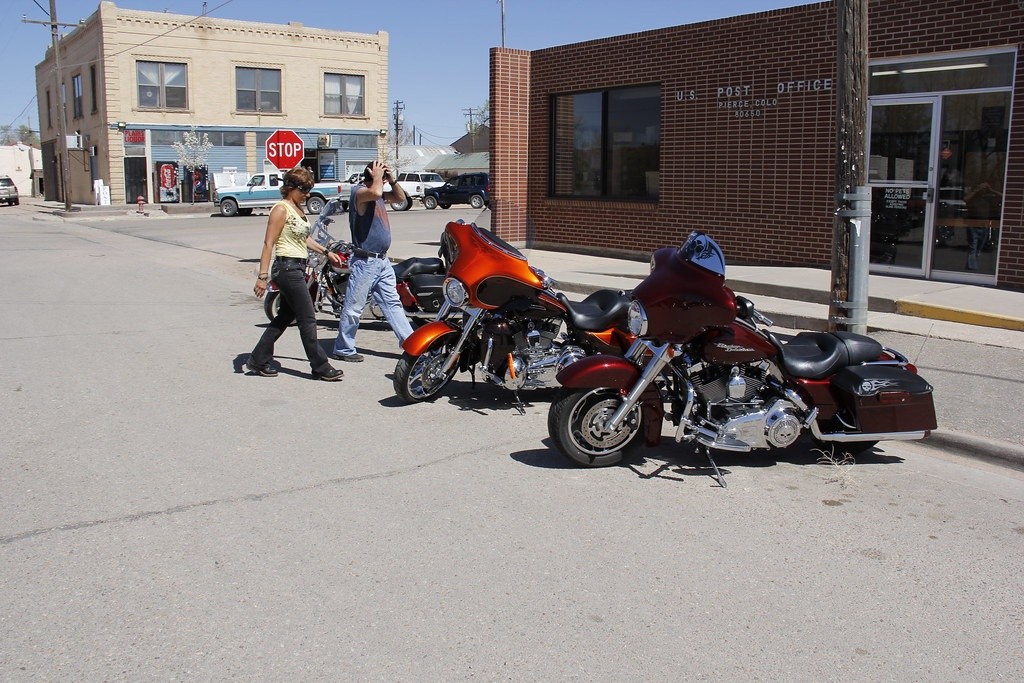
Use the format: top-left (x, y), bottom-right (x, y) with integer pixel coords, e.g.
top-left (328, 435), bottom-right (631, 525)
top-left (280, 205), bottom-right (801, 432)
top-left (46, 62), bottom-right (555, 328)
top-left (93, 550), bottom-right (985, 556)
top-left (678, 230), bottom-right (726, 276)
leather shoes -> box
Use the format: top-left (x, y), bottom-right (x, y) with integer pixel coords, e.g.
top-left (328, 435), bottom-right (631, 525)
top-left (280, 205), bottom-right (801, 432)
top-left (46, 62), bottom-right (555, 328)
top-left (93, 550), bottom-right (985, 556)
top-left (312, 368), bottom-right (344, 380)
top-left (247, 359), bottom-right (278, 376)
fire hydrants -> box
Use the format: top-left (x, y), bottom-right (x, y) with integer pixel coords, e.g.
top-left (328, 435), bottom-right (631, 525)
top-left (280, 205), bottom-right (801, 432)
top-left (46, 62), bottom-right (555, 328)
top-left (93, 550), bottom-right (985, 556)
top-left (137, 196), bottom-right (146, 213)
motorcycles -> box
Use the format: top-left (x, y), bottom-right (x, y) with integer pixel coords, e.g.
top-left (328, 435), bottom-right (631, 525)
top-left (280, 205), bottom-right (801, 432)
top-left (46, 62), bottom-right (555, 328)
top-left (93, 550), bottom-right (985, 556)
top-left (264, 197), bottom-right (463, 328)
top-left (392, 219), bottom-right (676, 404)
top-left (547, 231), bottom-right (938, 488)
top-left (983, 202), bottom-right (1001, 252)
top-left (869, 221), bottom-right (897, 266)
top-left (932, 201), bottom-right (954, 247)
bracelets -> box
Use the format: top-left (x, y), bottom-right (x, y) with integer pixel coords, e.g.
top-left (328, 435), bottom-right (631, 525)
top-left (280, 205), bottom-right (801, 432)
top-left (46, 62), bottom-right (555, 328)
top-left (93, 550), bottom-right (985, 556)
top-left (324, 250), bottom-right (330, 256)
top-left (258, 273), bottom-right (268, 280)
top-left (389, 180), bottom-right (397, 185)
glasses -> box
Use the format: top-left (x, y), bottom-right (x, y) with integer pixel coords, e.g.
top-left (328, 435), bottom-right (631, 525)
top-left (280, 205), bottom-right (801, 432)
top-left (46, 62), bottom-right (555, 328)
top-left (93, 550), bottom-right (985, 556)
top-left (289, 179), bottom-right (311, 193)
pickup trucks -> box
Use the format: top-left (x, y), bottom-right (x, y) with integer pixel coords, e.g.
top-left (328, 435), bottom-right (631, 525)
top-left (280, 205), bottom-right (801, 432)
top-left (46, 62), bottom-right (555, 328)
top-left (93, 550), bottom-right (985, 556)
top-left (213, 172), bottom-right (352, 217)
top-left (340, 172), bottom-right (423, 212)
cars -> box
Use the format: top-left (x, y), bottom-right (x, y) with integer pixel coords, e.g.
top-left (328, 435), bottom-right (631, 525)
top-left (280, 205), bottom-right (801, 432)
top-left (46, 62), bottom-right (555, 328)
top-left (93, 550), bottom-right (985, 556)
top-left (342, 172), bottom-right (396, 212)
top-left (869, 174), bottom-right (924, 225)
top-left (382, 172), bottom-right (455, 201)
top-left (935, 187), bottom-right (968, 212)
top-left (421, 171), bottom-right (490, 210)
top-left (0, 175), bottom-right (20, 206)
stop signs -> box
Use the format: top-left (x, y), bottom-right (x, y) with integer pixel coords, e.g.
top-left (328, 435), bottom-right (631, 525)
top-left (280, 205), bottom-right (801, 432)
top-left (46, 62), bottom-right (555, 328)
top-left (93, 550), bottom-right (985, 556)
top-left (265, 129), bottom-right (305, 170)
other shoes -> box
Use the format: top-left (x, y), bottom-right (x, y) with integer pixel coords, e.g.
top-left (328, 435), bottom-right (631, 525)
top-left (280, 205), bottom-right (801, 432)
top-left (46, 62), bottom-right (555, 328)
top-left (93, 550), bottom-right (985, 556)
top-left (333, 350), bottom-right (364, 362)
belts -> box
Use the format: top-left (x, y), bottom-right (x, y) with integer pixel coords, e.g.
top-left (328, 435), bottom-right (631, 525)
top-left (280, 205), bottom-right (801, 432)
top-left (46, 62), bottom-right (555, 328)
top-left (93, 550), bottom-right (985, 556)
top-left (353, 248), bottom-right (385, 259)
top-left (277, 256), bottom-right (310, 267)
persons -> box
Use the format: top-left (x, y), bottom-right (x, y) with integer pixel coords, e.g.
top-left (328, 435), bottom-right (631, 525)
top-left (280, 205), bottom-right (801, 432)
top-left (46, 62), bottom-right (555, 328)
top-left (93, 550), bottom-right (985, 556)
top-left (307, 166), bottom-right (314, 179)
top-left (247, 169), bottom-right (344, 380)
top-left (331, 160), bottom-right (414, 363)
top-left (965, 178), bottom-right (1002, 271)
